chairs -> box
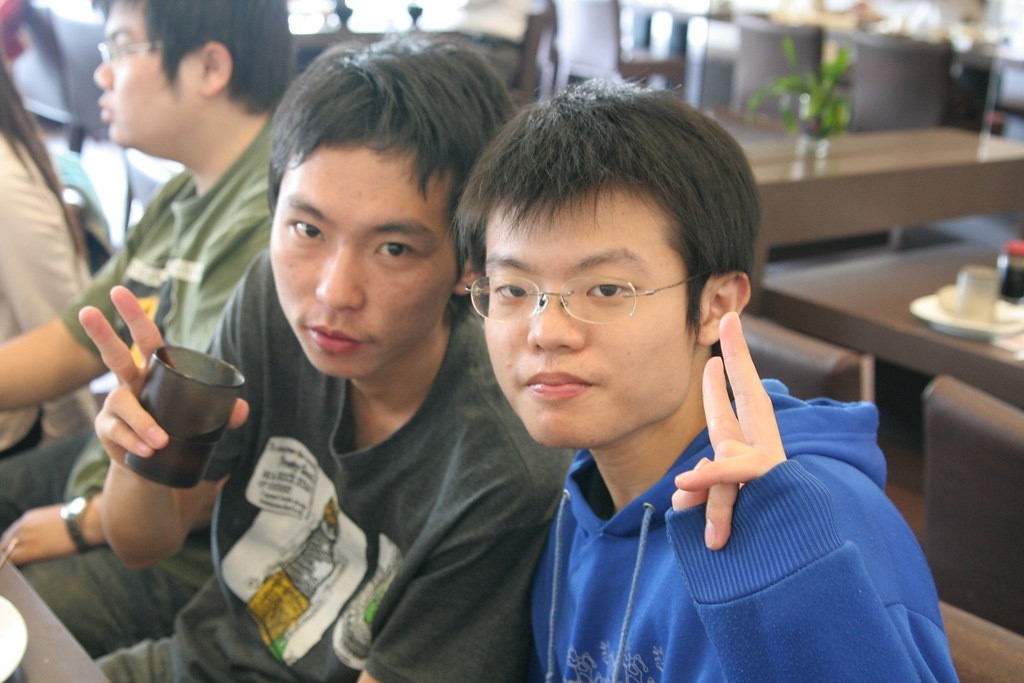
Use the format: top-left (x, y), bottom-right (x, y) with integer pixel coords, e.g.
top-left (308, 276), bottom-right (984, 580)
top-left (547, 1), bottom-right (687, 106)
top-left (737, 313), bottom-right (1024, 682)
top-left (724, 20), bottom-right (991, 246)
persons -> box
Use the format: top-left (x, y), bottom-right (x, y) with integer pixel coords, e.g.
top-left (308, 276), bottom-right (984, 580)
top-left (78, 31), bottom-right (580, 683)
top-left (0, 3), bottom-right (99, 460)
top-left (0, 0), bottom-right (297, 661)
top-left (443, 77), bottom-right (956, 683)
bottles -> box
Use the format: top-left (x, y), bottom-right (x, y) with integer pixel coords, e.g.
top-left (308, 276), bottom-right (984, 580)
top-left (996, 240), bottom-right (1024, 301)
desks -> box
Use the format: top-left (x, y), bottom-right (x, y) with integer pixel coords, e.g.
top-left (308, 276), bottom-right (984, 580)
top-left (727, 231), bottom-right (1023, 418)
top-left (735, 127), bottom-right (1024, 312)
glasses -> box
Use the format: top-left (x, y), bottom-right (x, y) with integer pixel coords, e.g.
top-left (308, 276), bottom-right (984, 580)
top-left (465, 269), bottom-right (711, 325)
top-left (97, 41), bottom-right (165, 65)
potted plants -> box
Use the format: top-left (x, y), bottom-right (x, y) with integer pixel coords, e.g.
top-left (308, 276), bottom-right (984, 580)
top-left (748, 32), bottom-right (856, 160)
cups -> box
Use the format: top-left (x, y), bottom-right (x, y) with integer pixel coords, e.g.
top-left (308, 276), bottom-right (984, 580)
top-left (122, 346), bottom-right (246, 489)
top-left (952, 265), bottom-right (999, 323)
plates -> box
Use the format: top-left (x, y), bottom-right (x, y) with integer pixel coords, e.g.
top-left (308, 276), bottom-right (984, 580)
top-left (908, 294), bottom-right (1024, 341)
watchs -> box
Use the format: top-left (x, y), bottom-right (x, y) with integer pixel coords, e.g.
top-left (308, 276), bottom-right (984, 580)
top-left (61, 485), bottom-right (103, 552)
